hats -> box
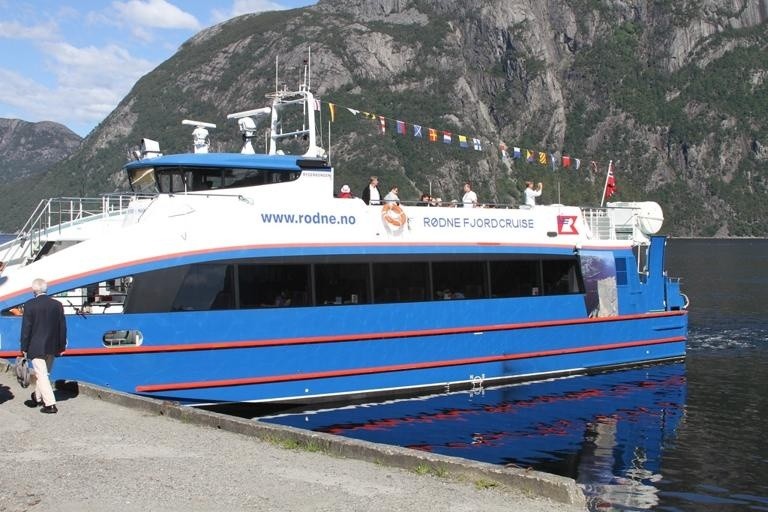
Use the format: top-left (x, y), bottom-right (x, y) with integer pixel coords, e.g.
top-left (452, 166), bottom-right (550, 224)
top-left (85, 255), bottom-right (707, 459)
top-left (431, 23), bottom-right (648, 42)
top-left (340, 184), bottom-right (350, 193)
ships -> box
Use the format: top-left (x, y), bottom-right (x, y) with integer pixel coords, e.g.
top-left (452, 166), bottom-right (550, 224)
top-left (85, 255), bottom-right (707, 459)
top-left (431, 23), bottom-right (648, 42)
top-left (0, 54), bottom-right (689, 406)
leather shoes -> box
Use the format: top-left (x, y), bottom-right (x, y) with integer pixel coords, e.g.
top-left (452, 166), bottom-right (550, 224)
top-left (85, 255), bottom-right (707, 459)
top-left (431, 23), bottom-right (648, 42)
top-left (31, 391), bottom-right (42, 404)
top-left (40, 404), bottom-right (57, 413)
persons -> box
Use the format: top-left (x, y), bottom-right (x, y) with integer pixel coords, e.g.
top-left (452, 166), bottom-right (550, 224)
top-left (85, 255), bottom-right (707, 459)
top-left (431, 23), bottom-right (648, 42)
top-left (523, 180), bottom-right (543, 207)
top-left (474, 199), bottom-right (513, 209)
top-left (361, 175), bottom-right (382, 206)
top-left (19, 278), bottom-right (68, 413)
top-left (462, 183), bottom-right (476, 208)
top-left (415, 193), bottom-right (458, 208)
top-left (336, 184), bottom-right (353, 198)
top-left (383, 185), bottom-right (402, 206)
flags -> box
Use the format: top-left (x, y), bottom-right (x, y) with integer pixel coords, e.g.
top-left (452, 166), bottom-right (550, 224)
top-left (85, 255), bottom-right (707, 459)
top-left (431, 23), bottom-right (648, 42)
top-left (605, 162), bottom-right (617, 200)
top-left (311, 98), bottom-right (599, 173)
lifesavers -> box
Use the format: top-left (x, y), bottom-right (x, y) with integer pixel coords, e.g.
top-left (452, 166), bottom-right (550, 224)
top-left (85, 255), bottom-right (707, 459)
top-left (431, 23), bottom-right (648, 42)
top-left (381, 203), bottom-right (405, 226)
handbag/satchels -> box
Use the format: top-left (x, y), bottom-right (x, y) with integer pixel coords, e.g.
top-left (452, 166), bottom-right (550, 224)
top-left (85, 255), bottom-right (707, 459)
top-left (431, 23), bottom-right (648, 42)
top-left (15, 352), bottom-right (30, 388)
